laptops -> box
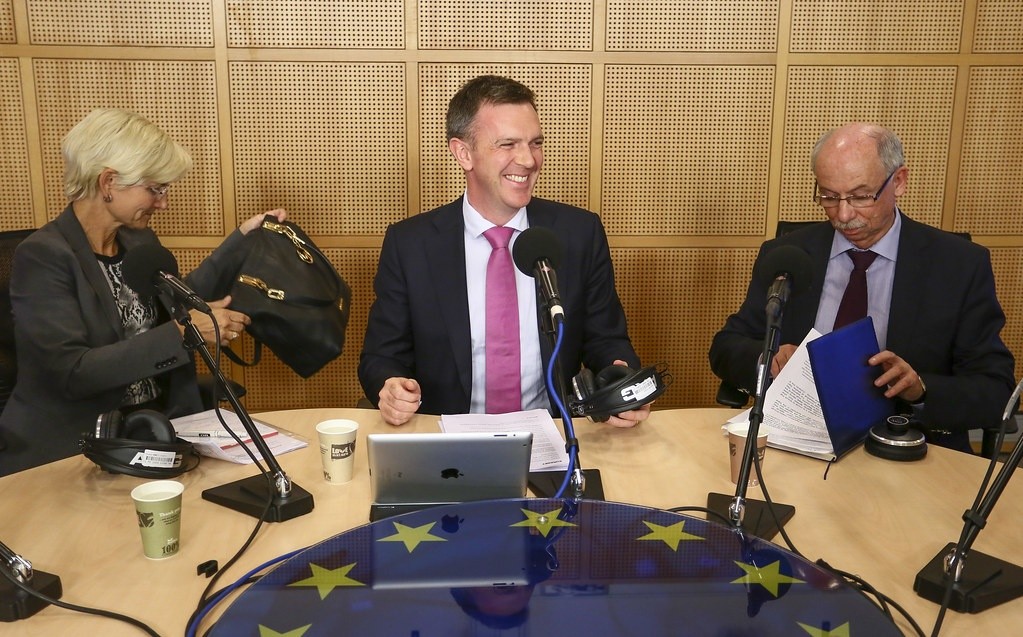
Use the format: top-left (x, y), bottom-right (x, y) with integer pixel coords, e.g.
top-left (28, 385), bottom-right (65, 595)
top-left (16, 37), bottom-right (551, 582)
top-left (366, 432), bottom-right (534, 504)
top-left (370, 498), bottom-right (528, 591)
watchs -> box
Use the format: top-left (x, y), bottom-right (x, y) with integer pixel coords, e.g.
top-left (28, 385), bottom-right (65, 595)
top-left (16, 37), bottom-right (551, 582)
top-left (907, 375), bottom-right (927, 404)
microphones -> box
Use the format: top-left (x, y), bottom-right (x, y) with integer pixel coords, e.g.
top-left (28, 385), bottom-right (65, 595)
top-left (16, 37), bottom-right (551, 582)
top-left (122, 242), bottom-right (212, 316)
top-left (759, 246), bottom-right (814, 320)
top-left (513, 228), bottom-right (564, 324)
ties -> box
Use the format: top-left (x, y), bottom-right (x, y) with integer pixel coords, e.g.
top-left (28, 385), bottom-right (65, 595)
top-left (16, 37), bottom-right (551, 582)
top-left (482, 227), bottom-right (520, 413)
top-left (833, 250), bottom-right (877, 334)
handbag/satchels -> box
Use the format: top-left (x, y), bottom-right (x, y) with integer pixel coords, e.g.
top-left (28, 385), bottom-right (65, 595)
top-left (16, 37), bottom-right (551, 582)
top-left (216, 215), bottom-right (351, 379)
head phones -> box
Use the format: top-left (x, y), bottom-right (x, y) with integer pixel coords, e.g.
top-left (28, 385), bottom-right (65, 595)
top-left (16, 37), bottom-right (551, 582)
top-left (569, 361), bottom-right (673, 423)
top-left (79, 408), bottom-right (194, 479)
top-left (864, 412), bottom-right (935, 462)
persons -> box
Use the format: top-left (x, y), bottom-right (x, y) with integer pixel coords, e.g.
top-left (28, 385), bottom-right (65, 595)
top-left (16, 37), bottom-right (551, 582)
top-left (0, 108), bottom-right (288, 477)
top-left (707, 123), bottom-right (1019, 453)
top-left (355, 76), bottom-right (658, 429)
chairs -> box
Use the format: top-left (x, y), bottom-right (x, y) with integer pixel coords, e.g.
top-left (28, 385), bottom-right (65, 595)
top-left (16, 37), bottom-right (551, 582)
top-left (0, 228), bottom-right (247, 477)
top-left (716, 220), bottom-right (1020, 460)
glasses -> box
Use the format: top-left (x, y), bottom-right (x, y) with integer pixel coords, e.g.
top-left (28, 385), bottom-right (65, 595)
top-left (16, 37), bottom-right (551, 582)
top-left (812, 166), bottom-right (899, 206)
top-left (141, 182), bottom-right (170, 200)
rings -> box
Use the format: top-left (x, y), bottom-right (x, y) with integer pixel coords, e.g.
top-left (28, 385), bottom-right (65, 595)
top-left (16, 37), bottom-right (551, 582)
top-left (232, 332), bottom-right (239, 338)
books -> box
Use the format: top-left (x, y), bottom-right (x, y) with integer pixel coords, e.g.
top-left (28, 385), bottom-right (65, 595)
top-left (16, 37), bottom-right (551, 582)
top-left (719, 313), bottom-right (898, 463)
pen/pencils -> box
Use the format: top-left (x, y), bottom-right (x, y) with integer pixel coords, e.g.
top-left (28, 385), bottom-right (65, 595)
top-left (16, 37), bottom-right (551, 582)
top-left (175, 432), bottom-right (250, 438)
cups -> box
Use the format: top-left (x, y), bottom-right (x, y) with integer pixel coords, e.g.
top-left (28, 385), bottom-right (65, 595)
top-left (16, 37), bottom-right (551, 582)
top-left (130, 480), bottom-right (185, 561)
top-left (726, 423), bottom-right (773, 488)
top-left (316, 419), bottom-right (360, 485)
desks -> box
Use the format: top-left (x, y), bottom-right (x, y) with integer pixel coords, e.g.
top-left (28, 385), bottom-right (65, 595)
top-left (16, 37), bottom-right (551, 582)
top-left (0, 408), bottom-right (1023, 637)
